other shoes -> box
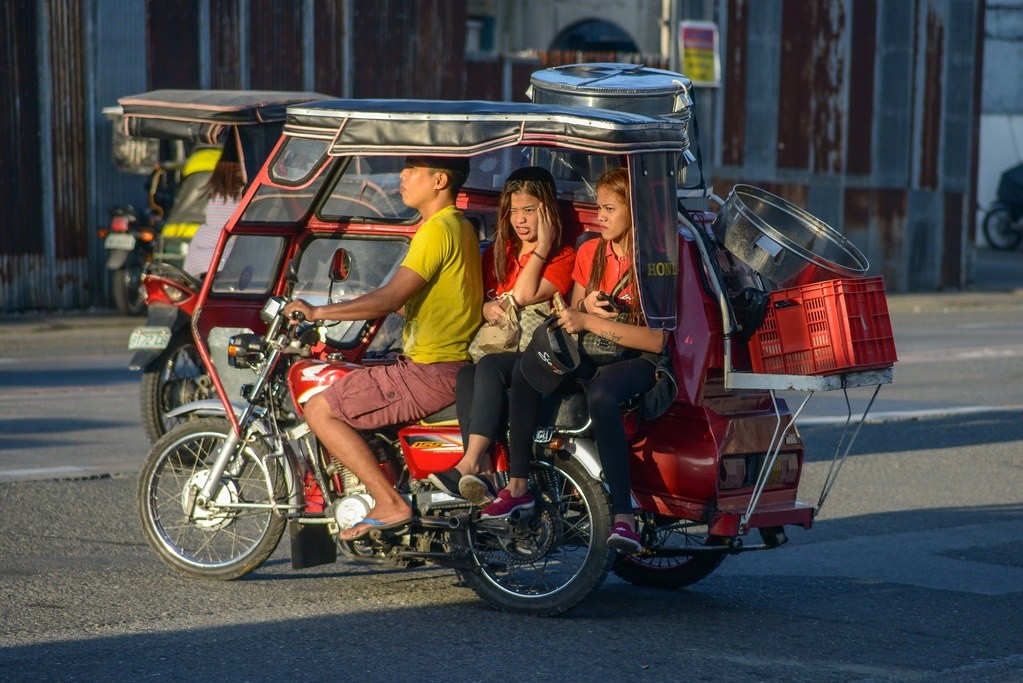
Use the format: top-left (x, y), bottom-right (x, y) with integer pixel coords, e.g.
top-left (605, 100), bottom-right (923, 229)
top-left (479, 488), bottom-right (536, 519)
top-left (606, 521), bottom-right (641, 554)
top-left (459, 474), bottom-right (498, 508)
top-left (428, 467), bottom-right (468, 500)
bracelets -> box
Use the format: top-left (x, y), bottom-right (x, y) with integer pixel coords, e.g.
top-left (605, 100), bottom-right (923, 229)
top-left (531, 251), bottom-right (546, 262)
top-left (577, 299), bottom-right (584, 310)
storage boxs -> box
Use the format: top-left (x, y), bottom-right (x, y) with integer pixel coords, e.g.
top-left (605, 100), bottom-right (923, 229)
top-left (748, 276), bottom-right (898, 376)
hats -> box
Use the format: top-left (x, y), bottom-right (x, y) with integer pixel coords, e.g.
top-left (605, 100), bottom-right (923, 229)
top-left (520, 308), bottom-right (580, 395)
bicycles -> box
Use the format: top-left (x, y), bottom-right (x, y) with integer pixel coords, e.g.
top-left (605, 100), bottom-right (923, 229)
top-left (981, 198), bottom-right (1023, 252)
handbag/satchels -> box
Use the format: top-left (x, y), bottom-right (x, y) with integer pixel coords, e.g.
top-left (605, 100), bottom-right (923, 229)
top-left (578, 312), bottom-right (631, 364)
top-left (515, 300), bottom-right (551, 352)
top-left (467, 306), bottom-right (520, 363)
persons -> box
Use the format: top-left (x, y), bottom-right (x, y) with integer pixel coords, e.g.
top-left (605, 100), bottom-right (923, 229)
top-left (428, 166), bottom-right (669, 552)
top-left (283, 156), bottom-right (484, 541)
top-left (184, 159), bottom-right (246, 281)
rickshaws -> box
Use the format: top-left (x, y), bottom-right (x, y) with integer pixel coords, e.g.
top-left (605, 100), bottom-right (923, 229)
top-left (136, 98), bottom-right (816, 617)
top-left (98, 90), bottom-right (361, 467)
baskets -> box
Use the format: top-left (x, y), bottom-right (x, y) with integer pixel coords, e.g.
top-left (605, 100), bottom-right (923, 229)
top-left (748, 273), bottom-right (899, 376)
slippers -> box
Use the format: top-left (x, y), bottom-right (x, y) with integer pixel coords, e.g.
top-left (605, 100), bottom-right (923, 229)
top-left (338, 515), bottom-right (413, 541)
